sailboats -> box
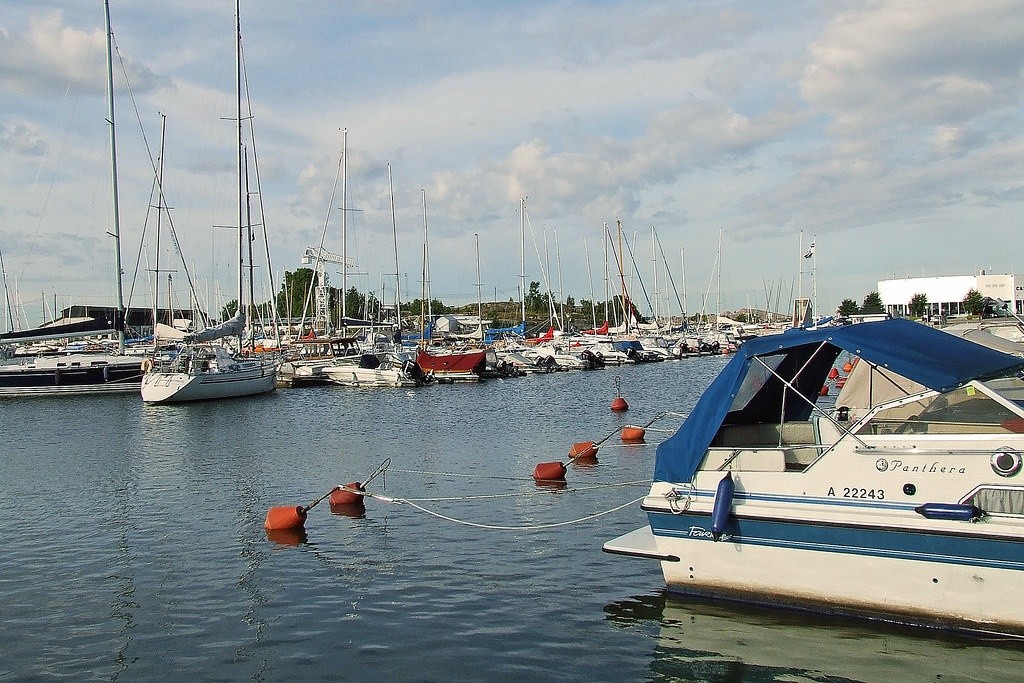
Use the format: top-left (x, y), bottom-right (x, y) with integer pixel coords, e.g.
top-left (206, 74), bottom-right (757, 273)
top-left (0, 1), bottom-right (847, 405)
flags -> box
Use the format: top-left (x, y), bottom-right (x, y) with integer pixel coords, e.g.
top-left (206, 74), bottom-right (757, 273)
top-left (804, 240), bottom-right (814, 259)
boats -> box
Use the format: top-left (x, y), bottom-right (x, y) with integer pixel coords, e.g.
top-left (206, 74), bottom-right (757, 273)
top-left (600, 319), bottom-right (1023, 647)
